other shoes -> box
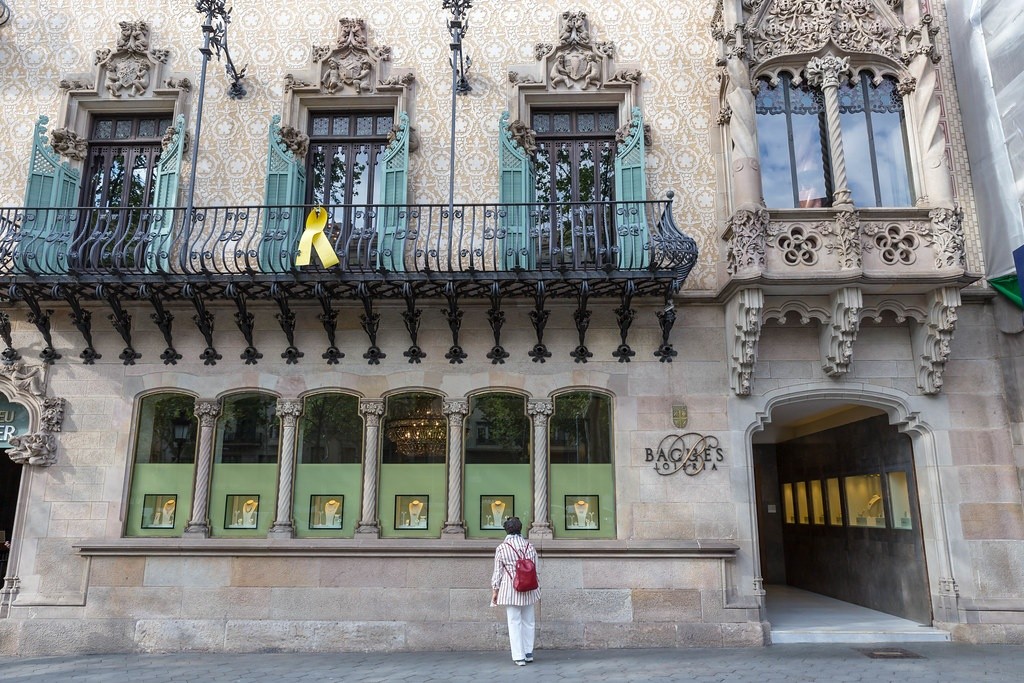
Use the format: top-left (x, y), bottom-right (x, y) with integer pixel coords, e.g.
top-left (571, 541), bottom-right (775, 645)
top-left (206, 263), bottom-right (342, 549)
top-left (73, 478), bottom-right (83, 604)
top-left (515, 659), bottom-right (526, 666)
top-left (525, 653), bottom-right (534, 661)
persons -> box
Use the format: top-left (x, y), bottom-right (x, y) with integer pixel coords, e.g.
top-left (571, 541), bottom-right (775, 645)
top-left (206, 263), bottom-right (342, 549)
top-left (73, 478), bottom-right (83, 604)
top-left (509, 10), bottom-right (641, 157)
top-left (277, 15), bottom-right (419, 157)
top-left (57, 18), bottom-right (193, 161)
top-left (490, 516), bottom-right (541, 666)
top-left (12, 363), bottom-right (46, 396)
top-left (4, 434), bottom-right (57, 467)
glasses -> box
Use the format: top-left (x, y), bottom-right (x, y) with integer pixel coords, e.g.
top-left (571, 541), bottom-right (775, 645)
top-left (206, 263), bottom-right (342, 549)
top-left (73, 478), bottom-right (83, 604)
top-left (506, 516), bottom-right (520, 521)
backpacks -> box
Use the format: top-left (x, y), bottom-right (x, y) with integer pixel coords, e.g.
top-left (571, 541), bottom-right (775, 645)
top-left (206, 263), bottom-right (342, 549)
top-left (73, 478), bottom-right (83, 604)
top-left (501, 542), bottom-right (538, 592)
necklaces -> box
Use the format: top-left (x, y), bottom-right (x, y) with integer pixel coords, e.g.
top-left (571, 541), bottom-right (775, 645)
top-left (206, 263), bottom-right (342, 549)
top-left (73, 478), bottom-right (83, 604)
top-left (165, 503), bottom-right (175, 514)
top-left (576, 503), bottom-right (587, 513)
top-left (411, 503), bottom-right (421, 515)
top-left (245, 502), bottom-right (254, 513)
top-left (326, 502), bottom-right (339, 512)
top-left (495, 504), bottom-right (501, 513)
